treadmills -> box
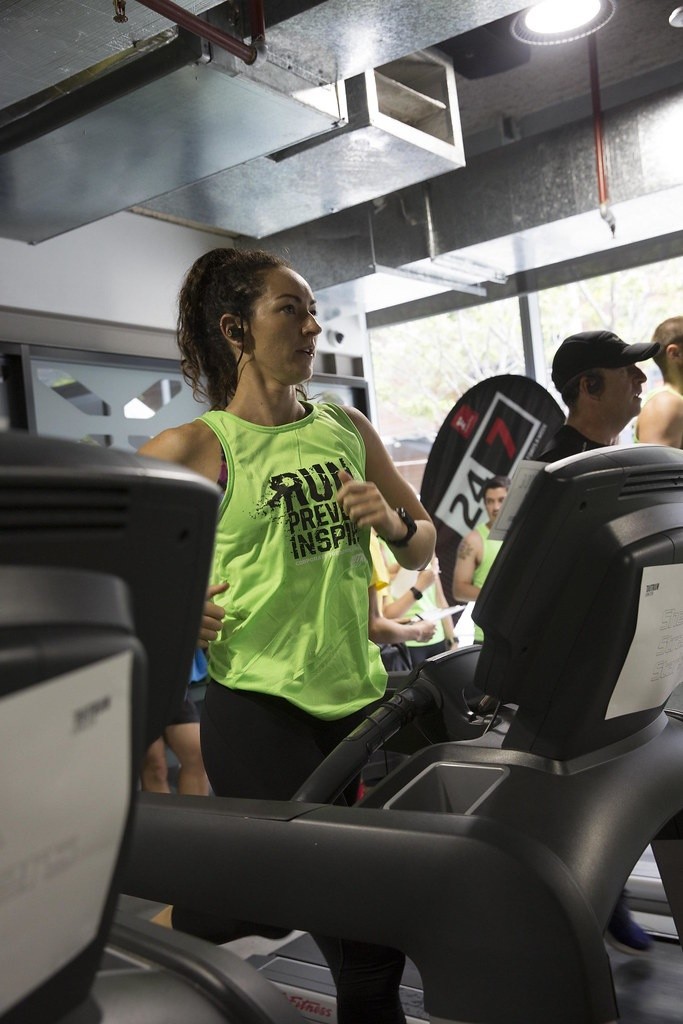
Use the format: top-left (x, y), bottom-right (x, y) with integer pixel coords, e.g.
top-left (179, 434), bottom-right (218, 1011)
top-left (0, 435), bottom-right (683, 1024)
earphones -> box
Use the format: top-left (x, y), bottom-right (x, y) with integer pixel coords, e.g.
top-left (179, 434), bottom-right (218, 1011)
top-left (230, 327), bottom-right (243, 339)
top-left (586, 374), bottom-right (605, 397)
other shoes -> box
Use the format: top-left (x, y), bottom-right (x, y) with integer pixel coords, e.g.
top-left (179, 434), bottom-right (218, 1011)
top-left (605, 890), bottom-right (654, 955)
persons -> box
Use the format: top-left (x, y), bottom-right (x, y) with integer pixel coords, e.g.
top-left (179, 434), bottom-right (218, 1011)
top-left (138, 249), bottom-right (438, 1024)
top-left (633, 316), bottom-right (683, 450)
top-left (368, 529), bottom-right (459, 672)
top-left (452, 476), bottom-right (512, 644)
top-left (538, 332), bottom-right (660, 463)
top-left (139, 693), bottom-right (209, 795)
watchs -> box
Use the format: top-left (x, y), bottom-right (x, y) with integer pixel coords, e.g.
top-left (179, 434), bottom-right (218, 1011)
top-left (376, 508), bottom-right (417, 548)
top-left (410, 587), bottom-right (422, 600)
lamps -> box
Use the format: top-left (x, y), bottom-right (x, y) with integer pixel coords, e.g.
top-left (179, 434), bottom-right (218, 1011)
top-left (508, 1), bottom-right (615, 45)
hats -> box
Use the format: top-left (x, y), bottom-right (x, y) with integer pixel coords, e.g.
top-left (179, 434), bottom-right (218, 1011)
top-left (552, 331), bottom-right (660, 393)
